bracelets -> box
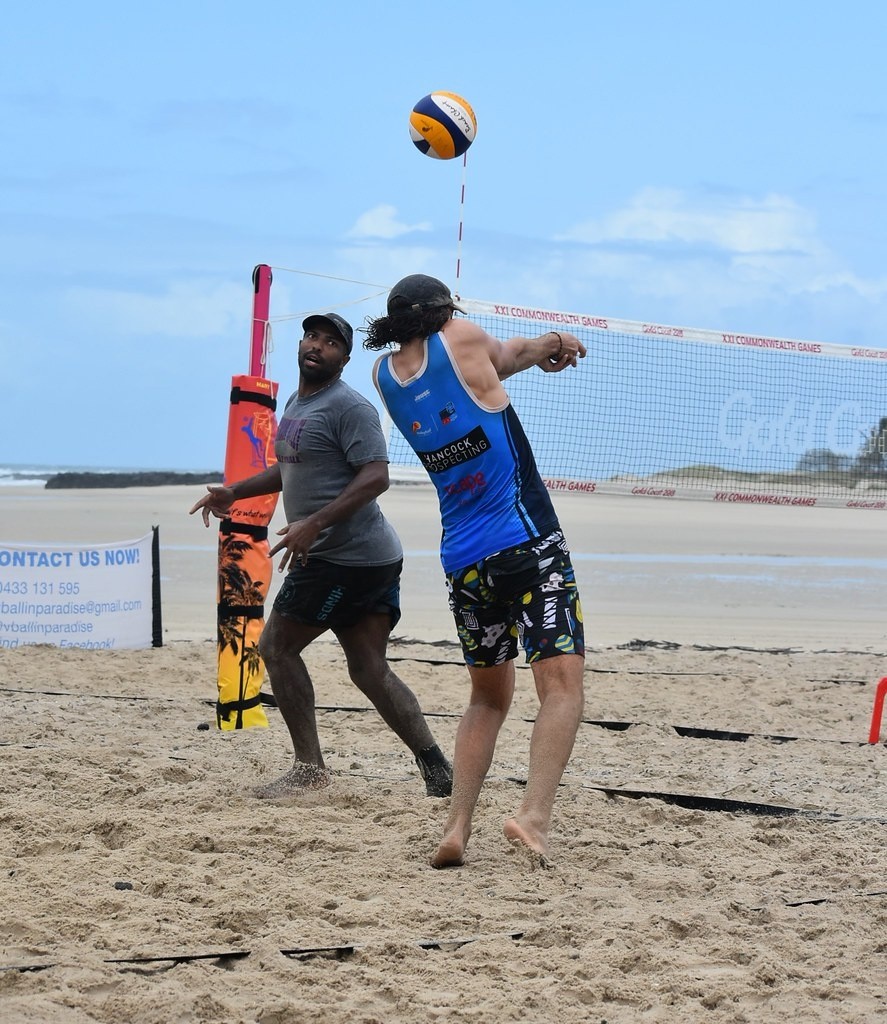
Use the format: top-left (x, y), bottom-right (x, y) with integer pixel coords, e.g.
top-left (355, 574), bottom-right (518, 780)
top-left (550, 332), bottom-right (563, 359)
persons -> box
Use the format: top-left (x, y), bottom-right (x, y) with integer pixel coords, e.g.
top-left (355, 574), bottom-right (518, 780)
top-left (358, 274), bottom-right (589, 869)
top-left (189, 313), bottom-right (453, 800)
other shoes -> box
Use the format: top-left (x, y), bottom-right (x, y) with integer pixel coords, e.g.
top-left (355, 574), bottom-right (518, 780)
top-left (415, 744), bottom-right (453, 798)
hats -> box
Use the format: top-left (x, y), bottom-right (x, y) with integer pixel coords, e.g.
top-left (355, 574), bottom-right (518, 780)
top-left (388, 273), bottom-right (468, 316)
top-left (302, 313), bottom-right (353, 352)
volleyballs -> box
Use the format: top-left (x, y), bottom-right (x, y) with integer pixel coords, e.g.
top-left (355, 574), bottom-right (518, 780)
top-left (407, 90), bottom-right (478, 161)
